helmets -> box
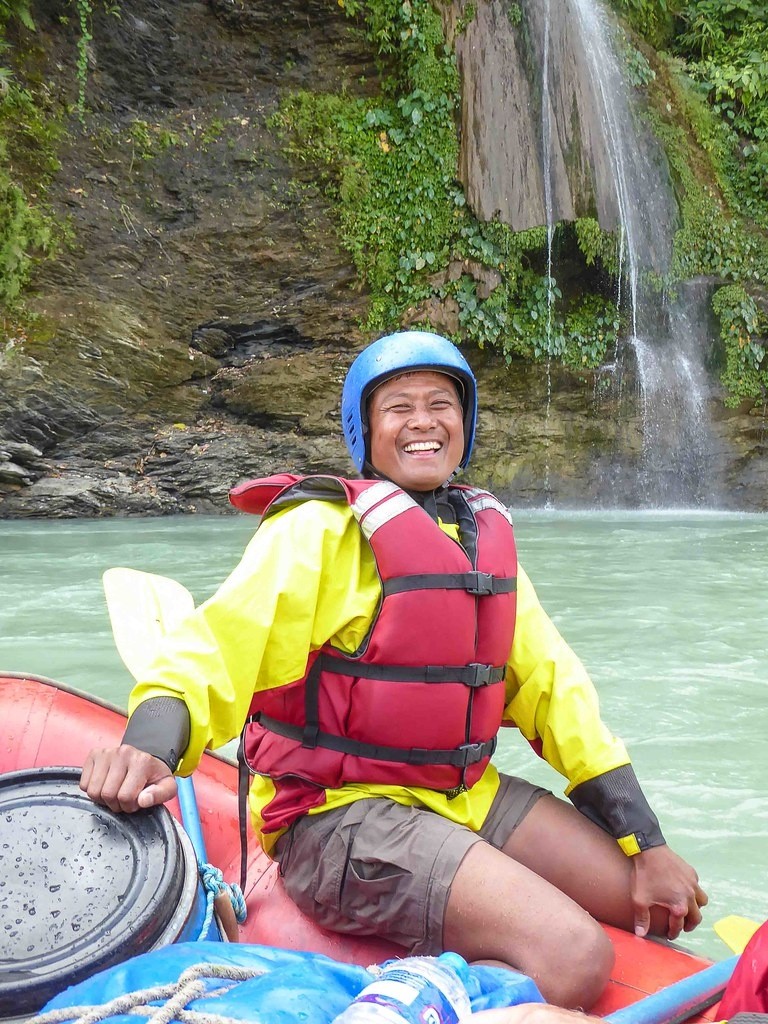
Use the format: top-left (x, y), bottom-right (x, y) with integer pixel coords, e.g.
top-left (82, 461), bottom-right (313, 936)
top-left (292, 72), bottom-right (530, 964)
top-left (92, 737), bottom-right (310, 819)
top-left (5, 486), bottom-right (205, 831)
top-left (340, 329), bottom-right (477, 474)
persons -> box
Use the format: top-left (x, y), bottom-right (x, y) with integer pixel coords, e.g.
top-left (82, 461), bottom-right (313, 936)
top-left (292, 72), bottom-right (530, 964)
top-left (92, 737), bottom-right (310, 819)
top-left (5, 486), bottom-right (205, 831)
top-left (79, 331), bottom-right (707, 1010)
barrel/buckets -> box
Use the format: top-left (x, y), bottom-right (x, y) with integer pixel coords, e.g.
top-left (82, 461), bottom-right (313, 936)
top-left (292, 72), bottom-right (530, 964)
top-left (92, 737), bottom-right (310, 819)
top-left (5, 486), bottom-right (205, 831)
top-left (0, 766), bottom-right (229, 1023)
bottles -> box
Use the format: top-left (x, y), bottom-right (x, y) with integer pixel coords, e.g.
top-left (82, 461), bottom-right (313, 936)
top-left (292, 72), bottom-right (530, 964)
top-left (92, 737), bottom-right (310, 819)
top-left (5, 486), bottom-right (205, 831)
top-left (330, 952), bottom-right (472, 1024)
